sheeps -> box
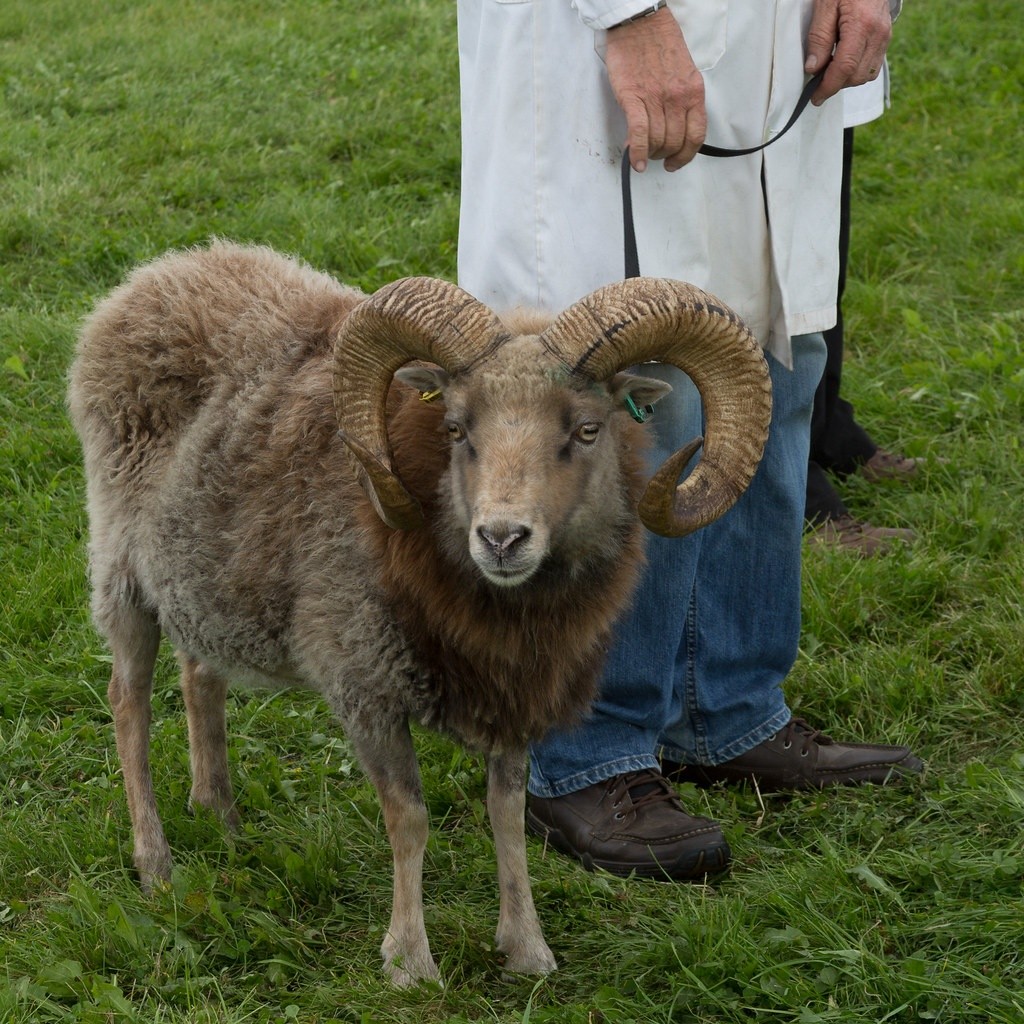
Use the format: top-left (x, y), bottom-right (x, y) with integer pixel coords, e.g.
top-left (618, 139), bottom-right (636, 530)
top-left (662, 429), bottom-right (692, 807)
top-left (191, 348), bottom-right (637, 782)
top-left (54, 227), bottom-right (781, 1004)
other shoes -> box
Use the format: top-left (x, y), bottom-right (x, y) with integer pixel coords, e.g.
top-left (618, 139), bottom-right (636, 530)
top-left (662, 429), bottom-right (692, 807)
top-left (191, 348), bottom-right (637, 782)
top-left (859, 448), bottom-right (950, 483)
top-left (808, 512), bottom-right (914, 560)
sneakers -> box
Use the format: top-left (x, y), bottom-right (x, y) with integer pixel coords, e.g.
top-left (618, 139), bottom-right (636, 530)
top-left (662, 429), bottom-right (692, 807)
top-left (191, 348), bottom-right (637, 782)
top-left (524, 766), bottom-right (731, 886)
top-left (653, 714), bottom-right (922, 789)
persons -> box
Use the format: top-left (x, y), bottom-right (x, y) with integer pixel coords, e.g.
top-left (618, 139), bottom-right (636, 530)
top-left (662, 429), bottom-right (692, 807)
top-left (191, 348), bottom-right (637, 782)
top-left (803, 128), bottom-right (929, 559)
top-left (452, 0), bottom-right (916, 886)
